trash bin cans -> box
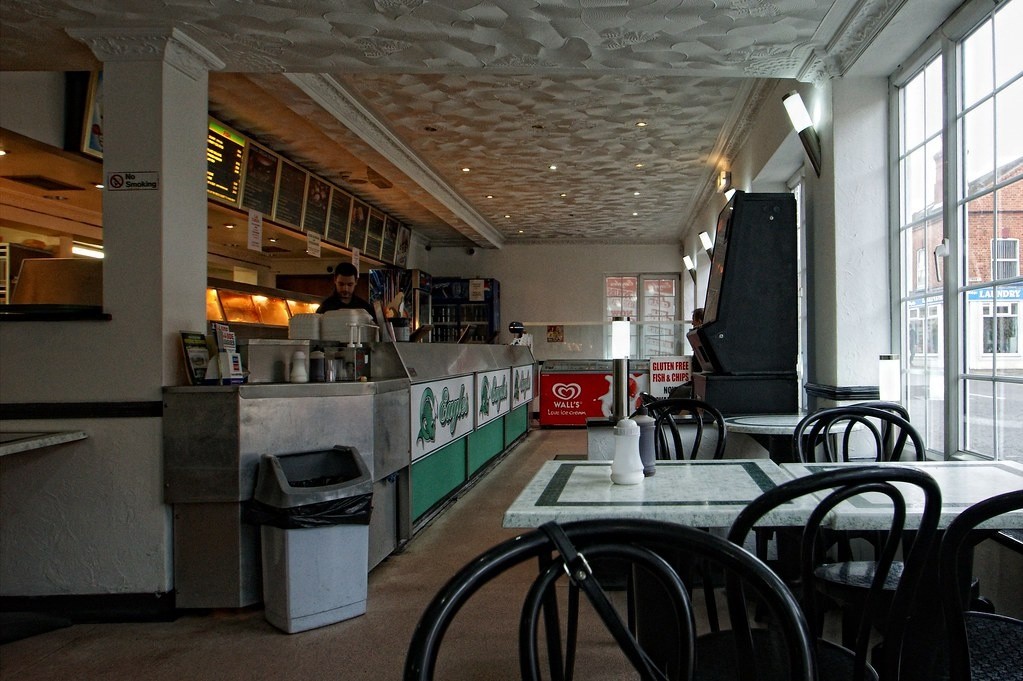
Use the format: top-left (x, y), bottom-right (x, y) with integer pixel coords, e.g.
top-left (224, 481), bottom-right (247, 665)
top-left (253, 445), bottom-right (372, 634)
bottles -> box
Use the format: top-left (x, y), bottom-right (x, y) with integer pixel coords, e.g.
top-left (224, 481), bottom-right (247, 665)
top-left (610, 416), bottom-right (645, 484)
top-left (630, 404), bottom-right (656, 477)
top-left (310, 344), bottom-right (326, 383)
top-left (289, 351), bottom-right (307, 383)
top-left (430, 305), bottom-right (491, 343)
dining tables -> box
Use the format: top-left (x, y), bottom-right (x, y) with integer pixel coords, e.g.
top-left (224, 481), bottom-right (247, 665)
top-left (712, 413), bottom-right (865, 457)
top-left (0, 430), bottom-right (89, 457)
top-left (502, 456), bottom-right (1023, 680)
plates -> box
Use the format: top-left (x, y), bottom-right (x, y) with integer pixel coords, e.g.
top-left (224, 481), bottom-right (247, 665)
top-left (288, 308), bottom-right (374, 342)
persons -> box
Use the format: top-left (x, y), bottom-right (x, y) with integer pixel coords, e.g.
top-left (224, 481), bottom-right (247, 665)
top-left (315, 263), bottom-right (374, 320)
top-left (690, 309), bottom-right (703, 372)
top-left (909, 325), bottom-right (918, 368)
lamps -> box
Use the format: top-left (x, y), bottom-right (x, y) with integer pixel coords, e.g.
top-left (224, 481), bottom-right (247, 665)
top-left (95, 184), bottom-right (105, 188)
top-left (635, 164), bottom-right (643, 167)
top-left (560, 193), bottom-right (566, 197)
top-left (223, 223), bottom-right (236, 228)
top-left (486, 195), bottom-right (494, 199)
top-left (518, 230), bottom-right (524, 233)
top-left (635, 122), bottom-right (647, 128)
top-left (549, 165), bottom-right (556, 169)
top-left (634, 192), bottom-right (640, 196)
top-left (269, 238), bottom-right (278, 242)
top-left (462, 167), bottom-right (470, 171)
top-left (0, 150), bottom-right (6, 156)
top-left (632, 213), bottom-right (637, 216)
top-left (504, 215), bottom-right (511, 218)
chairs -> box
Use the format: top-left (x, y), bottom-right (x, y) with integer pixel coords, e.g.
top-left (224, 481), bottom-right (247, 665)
top-left (629, 399), bottom-right (728, 461)
top-left (793, 405), bottom-right (928, 651)
top-left (402, 517), bottom-right (817, 681)
top-left (661, 466), bottom-right (942, 681)
top-left (871, 490), bottom-right (1023, 681)
